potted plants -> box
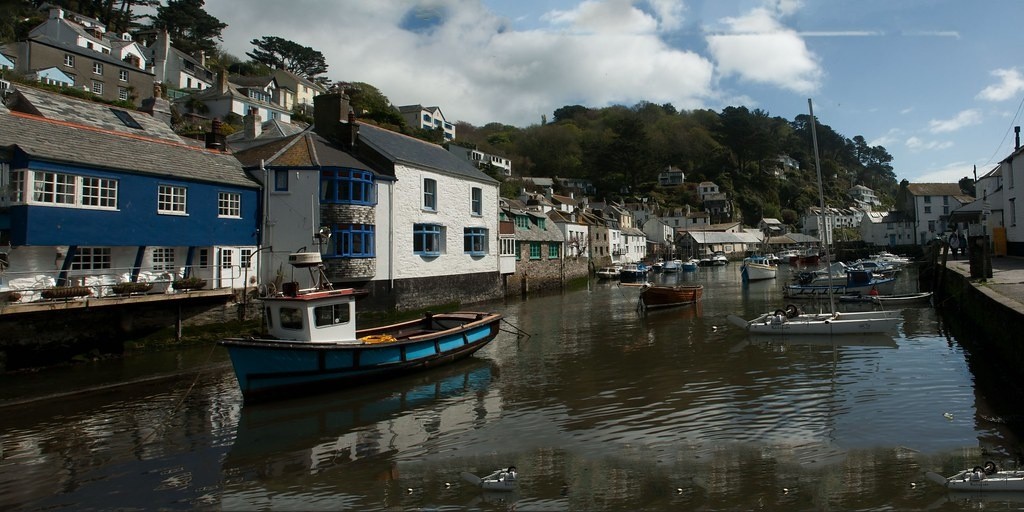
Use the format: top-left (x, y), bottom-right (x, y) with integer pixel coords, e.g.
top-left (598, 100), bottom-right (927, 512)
top-left (171, 277), bottom-right (209, 292)
top-left (111, 283), bottom-right (155, 298)
top-left (40, 287), bottom-right (92, 302)
top-left (0, 292), bottom-right (21, 305)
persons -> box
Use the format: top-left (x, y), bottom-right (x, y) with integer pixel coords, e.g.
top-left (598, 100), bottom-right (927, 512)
top-left (949, 231), bottom-right (959, 260)
top-left (959, 235), bottom-right (967, 256)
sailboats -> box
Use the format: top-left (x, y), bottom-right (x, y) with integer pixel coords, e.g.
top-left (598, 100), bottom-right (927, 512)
top-left (726, 98), bottom-right (905, 334)
top-left (726, 333), bottom-right (899, 381)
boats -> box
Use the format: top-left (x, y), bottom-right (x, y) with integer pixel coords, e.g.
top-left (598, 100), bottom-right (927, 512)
top-left (740, 254), bottom-right (776, 281)
top-left (637, 281), bottom-right (704, 312)
top-left (745, 243), bottom-right (820, 264)
top-left (220, 356), bottom-right (502, 473)
top-left (926, 460), bottom-right (1024, 490)
top-left (217, 246), bottom-right (505, 406)
top-left (596, 263), bottom-right (652, 281)
top-left (837, 291), bottom-right (934, 308)
top-left (653, 251), bottom-right (729, 273)
top-left (781, 250), bottom-right (916, 298)
